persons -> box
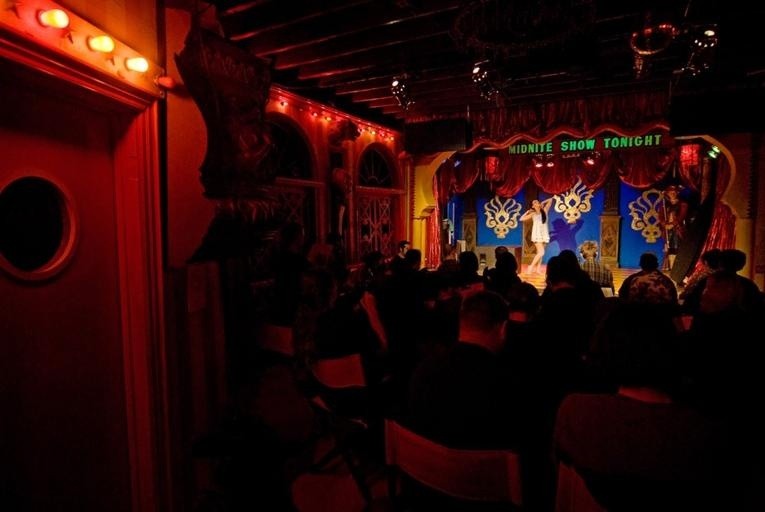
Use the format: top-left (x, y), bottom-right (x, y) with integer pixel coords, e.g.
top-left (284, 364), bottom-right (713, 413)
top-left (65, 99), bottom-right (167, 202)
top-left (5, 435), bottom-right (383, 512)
top-left (192, 221), bottom-right (765, 510)
top-left (328, 166), bottom-right (352, 255)
top-left (518, 196), bottom-right (553, 276)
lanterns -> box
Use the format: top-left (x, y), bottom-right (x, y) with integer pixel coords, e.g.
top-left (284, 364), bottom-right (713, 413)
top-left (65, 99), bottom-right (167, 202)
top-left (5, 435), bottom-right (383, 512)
top-left (484, 154), bottom-right (505, 194)
top-left (678, 140), bottom-right (700, 178)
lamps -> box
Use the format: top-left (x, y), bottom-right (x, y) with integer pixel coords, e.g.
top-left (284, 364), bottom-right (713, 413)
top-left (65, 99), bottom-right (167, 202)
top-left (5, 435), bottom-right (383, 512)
top-left (468, 60), bottom-right (499, 101)
top-left (387, 74), bottom-right (415, 110)
top-left (680, 23), bottom-right (720, 76)
top-left (36, 7), bottom-right (175, 90)
